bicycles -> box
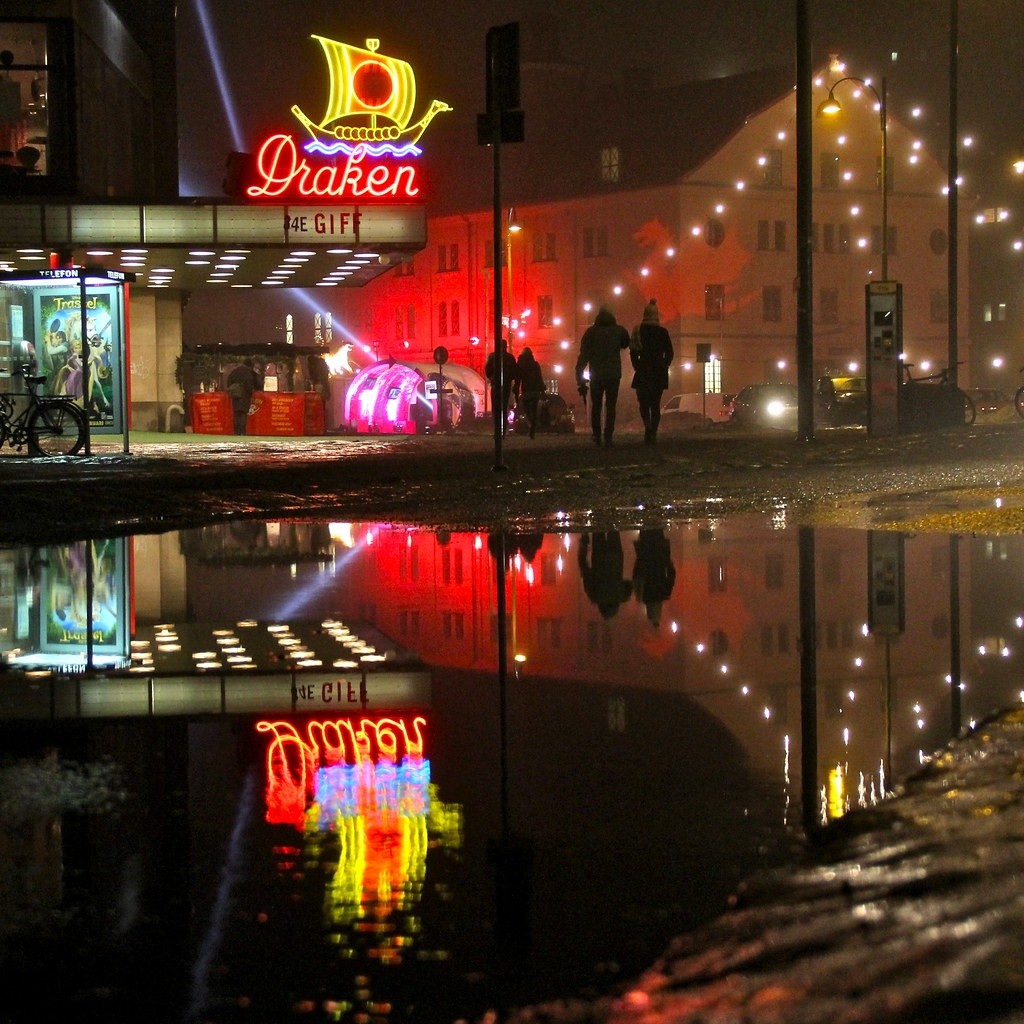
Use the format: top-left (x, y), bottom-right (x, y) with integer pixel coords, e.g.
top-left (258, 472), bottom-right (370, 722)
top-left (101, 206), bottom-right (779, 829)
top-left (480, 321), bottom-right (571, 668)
top-left (903, 361), bottom-right (977, 427)
top-left (0, 363), bottom-right (87, 457)
top-left (1014, 366), bottom-right (1024, 417)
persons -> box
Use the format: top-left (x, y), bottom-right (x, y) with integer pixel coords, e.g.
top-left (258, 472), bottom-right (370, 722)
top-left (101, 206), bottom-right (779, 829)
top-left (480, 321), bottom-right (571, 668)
top-left (231, 520), bottom-right (260, 550)
top-left (485, 340), bottom-right (517, 439)
top-left (512, 347), bottom-right (547, 439)
top-left (488, 532), bottom-right (544, 569)
top-left (227, 359), bottom-right (258, 436)
top-left (629, 298), bottom-right (674, 444)
top-left (575, 303), bottom-right (631, 449)
top-left (633, 529), bottom-right (677, 626)
top-left (577, 529), bottom-right (632, 619)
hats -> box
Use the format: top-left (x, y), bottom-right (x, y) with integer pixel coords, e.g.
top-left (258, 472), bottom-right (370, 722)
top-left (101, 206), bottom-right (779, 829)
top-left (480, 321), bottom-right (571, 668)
top-left (599, 303), bottom-right (616, 317)
top-left (643, 298), bottom-right (659, 320)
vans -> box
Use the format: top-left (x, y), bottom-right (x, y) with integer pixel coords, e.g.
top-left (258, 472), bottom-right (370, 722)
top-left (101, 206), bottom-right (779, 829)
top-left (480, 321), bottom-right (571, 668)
top-left (661, 393), bottom-right (737, 424)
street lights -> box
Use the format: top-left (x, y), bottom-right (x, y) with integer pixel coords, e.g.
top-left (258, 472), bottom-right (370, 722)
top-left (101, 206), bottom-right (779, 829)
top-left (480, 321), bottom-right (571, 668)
top-left (506, 205), bottom-right (522, 354)
top-left (510, 554), bottom-right (527, 680)
top-left (818, 72), bottom-right (891, 275)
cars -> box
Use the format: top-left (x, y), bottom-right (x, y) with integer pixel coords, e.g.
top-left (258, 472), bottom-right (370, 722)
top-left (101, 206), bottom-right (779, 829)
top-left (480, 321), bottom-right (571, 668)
top-left (514, 393), bottom-right (577, 435)
top-left (813, 372), bottom-right (869, 427)
top-left (960, 385), bottom-right (1014, 415)
top-left (729, 382), bottom-right (798, 426)
